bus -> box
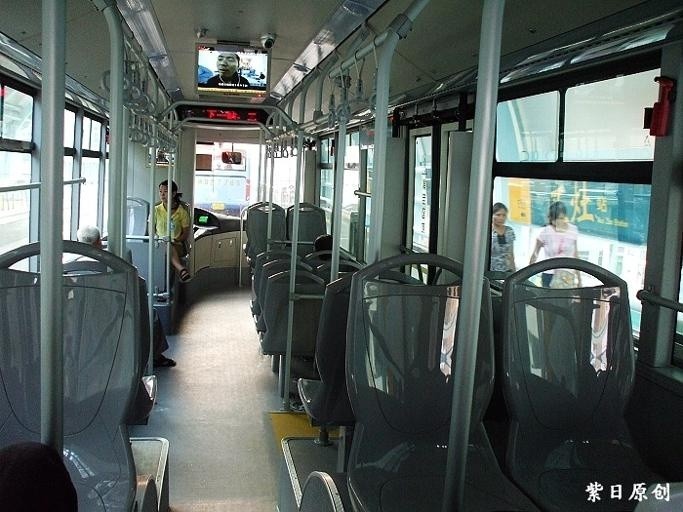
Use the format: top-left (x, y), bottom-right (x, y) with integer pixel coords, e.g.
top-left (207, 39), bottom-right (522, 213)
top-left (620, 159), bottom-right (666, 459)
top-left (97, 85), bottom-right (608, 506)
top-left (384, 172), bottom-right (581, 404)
top-left (495, 70), bottom-right (683, 345)
top-left (197, 144), bottom-right (250, 216)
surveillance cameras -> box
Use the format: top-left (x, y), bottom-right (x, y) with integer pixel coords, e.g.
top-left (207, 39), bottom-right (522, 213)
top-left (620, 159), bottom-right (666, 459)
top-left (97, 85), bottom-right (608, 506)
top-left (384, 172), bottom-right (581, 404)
top-left (260, 32), bottom-right (277, 51)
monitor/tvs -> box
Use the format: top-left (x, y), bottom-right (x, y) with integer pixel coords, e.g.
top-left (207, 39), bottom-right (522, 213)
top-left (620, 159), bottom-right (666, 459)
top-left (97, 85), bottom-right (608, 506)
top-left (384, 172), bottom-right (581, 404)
top-left (145, 146), bottom-right (178, 169)
top-left (192, 43), bottom-right (271, 104)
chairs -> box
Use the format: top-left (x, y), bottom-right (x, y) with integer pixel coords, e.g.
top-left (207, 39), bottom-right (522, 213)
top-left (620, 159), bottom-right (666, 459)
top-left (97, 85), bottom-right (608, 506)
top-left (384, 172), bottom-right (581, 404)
top-left (247, 202), bottom-right (674, 512)
top-left (0, 199), bottom-right (189, 511)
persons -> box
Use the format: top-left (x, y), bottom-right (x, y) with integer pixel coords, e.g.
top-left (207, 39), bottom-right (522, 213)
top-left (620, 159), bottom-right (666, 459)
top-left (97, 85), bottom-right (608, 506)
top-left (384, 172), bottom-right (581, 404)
top-left (67, 223), bottom-right (176, 367)
top-left (147, 178), bottom-right (191, 281)
top-left (489, 203), bottom-right (517, 272)
top-left (314, 234), bottom-right (333, 262)
top-left (0, 442), bottom-right (77, 512)
top-left (209, 52), bottom-right (249, 88)
top-left (528, 203), bottom-right (583, 288)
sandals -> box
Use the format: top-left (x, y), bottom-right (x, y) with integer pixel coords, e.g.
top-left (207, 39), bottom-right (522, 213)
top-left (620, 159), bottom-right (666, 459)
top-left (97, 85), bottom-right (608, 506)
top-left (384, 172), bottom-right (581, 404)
top-left (179, 269), bottom-right (190, 281)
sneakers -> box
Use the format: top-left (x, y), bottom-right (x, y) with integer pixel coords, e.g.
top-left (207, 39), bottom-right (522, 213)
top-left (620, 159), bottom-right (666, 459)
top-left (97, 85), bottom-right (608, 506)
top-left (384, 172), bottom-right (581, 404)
top-left (153, 355), bottom-right (176, 367)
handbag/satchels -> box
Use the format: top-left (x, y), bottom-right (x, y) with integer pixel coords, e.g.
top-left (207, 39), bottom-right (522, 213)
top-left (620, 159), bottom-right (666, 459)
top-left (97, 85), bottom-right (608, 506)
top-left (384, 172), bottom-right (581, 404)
top-left (549, 270), bottom-right (575, 289)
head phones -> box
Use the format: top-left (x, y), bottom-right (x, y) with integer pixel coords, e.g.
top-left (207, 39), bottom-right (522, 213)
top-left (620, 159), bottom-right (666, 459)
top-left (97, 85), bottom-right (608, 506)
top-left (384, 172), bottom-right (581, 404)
top-left (535, 433), bottom-right (651, 511)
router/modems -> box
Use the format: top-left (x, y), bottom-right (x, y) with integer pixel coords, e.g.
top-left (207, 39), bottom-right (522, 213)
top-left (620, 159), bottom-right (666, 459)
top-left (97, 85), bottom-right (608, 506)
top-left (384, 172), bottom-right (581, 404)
top-left (216, 40), bottom-right (250, 46)
